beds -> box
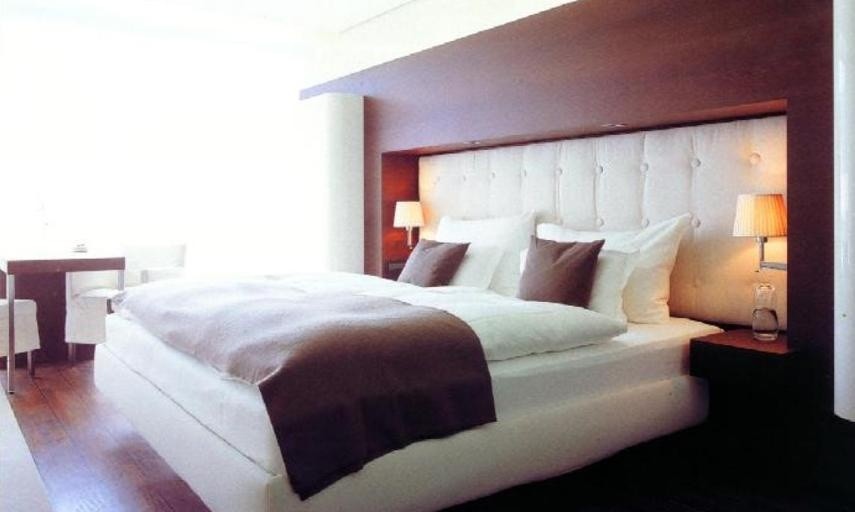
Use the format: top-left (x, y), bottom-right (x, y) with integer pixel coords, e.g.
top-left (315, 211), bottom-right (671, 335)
top-left (93, 286), bottom-right (729, 510)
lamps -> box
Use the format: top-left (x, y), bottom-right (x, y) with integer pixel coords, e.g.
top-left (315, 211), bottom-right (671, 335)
top-left (731, 193), bottom-right (787, 275)
top-left (392, 201), bottom-right (423, 249)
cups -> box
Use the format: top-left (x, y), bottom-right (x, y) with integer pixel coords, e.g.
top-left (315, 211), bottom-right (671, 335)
top-left (755, 282), bottom-right (775, 309)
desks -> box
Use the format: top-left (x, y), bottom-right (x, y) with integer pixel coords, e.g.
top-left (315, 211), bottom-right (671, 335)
top-left (689, 326), bottom-right (789, 420)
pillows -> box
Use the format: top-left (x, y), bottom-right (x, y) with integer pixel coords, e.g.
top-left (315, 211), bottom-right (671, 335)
top-left (395, 216), bottom-right (690, 327)
